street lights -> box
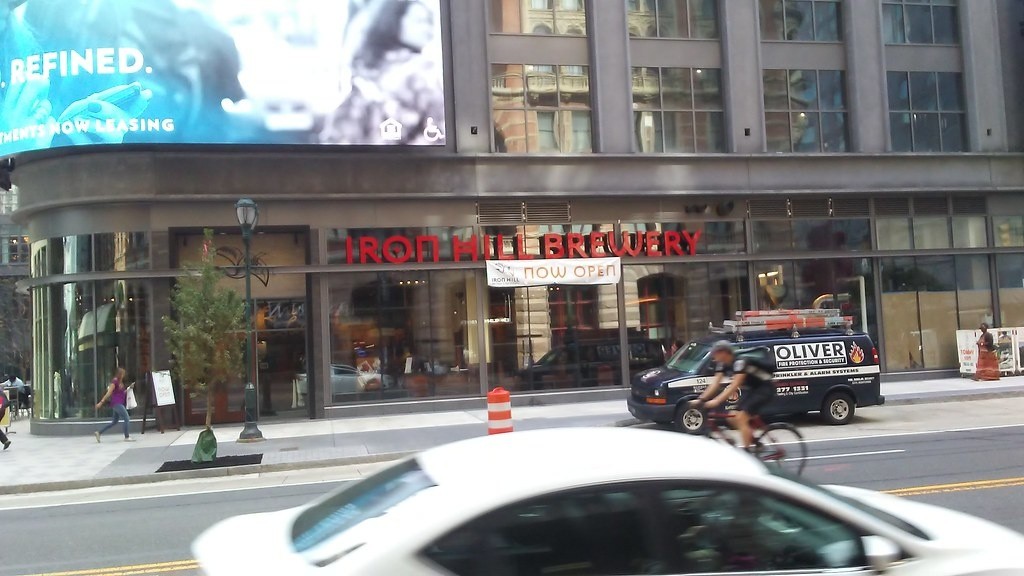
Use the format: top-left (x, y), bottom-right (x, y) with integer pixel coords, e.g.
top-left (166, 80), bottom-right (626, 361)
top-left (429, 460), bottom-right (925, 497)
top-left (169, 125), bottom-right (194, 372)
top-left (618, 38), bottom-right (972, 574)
top-left (233, 196), bottom-right (264, 440)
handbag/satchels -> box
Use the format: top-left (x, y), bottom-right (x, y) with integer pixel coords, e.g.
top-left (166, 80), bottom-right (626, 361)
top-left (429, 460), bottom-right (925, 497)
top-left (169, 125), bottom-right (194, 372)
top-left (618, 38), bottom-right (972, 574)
top-left (126, 386), bottom-right (137, 410)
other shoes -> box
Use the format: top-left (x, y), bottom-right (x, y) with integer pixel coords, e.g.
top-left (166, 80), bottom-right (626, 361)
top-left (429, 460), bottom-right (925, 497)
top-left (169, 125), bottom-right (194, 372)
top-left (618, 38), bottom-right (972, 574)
top-left (3, 441), bottom-right (11, 450)
top-left (95, 432), bottom-right (100, 443)
top-left (124, 438), bottom-right (136, 442)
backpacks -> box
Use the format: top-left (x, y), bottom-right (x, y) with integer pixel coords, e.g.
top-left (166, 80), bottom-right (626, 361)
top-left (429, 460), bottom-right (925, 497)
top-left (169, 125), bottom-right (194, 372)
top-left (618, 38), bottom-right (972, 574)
top-left (733, 346), bottom-right (778, 375)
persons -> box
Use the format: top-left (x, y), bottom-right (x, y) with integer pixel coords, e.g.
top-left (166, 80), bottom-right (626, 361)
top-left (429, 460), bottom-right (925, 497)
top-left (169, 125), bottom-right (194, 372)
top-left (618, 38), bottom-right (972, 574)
top-left (95, 368), bottom-right (136, 443)
top-left (0, 391), bottom-right (12, 450)
top-left (971, 323), bottom-right (1000, 381)
top-left (672, 340), bottom-right (684, 356)
top-left (0, 373), bottom-right (31, 417)
top-left (690, 340), bottom-right (776, 453)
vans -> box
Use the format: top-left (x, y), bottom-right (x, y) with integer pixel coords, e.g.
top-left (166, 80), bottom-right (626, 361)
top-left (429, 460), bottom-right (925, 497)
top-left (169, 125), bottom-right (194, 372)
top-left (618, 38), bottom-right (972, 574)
top-left (514, 338), bottom-right (666, 392)
top-left (627, 307), bottom-right (886, 436)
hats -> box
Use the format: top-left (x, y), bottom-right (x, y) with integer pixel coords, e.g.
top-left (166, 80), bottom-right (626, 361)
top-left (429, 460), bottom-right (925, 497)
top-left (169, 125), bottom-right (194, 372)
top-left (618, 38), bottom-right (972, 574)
top-left (711, 340), bottom-right (732, 352)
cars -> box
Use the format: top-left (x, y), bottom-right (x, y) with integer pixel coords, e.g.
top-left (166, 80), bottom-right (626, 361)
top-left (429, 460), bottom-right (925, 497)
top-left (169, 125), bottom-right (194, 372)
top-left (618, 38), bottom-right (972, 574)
top-left (299, 362), bottom-right (394, 404)
top-left (192, 422), bottom-right (1024, 575)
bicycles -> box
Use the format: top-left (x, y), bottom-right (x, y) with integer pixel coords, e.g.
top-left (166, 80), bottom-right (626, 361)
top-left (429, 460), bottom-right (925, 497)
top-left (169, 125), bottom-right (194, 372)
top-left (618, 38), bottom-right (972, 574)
top-left (0, 381), bottom-right (22, 426)
top-left (665, 400), bottom-right (810, 483)
